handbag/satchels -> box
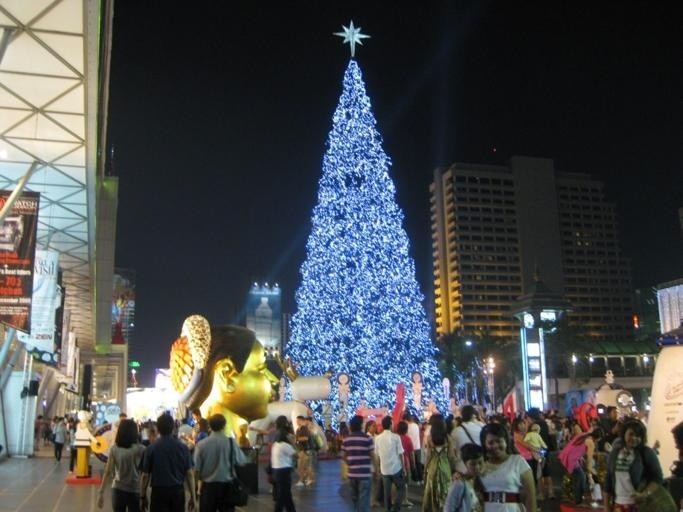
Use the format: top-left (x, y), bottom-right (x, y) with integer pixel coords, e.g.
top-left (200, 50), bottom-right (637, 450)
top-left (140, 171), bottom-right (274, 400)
top-left (635, 481), bottom-right (678, 512)
top-left (233, 479), bottom-right (248, 506)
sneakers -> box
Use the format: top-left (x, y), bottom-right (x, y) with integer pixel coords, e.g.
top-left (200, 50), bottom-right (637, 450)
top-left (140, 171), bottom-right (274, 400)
top-left (295, 479), bottom-right (312, 486)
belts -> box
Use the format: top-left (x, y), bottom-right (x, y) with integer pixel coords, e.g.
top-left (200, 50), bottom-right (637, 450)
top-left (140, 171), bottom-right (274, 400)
top-left (483, 491), bottom-right (521, 502)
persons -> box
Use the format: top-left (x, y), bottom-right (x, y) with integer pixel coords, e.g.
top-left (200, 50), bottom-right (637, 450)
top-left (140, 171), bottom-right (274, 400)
top-left (97, 407), bottom-right (246, 511)
top-left (169, 315), bottom-right (276, 447)
top-left (269, 405), bottom-right (682, 512)
top-left (35, 414), bottom-right (79, 474)
